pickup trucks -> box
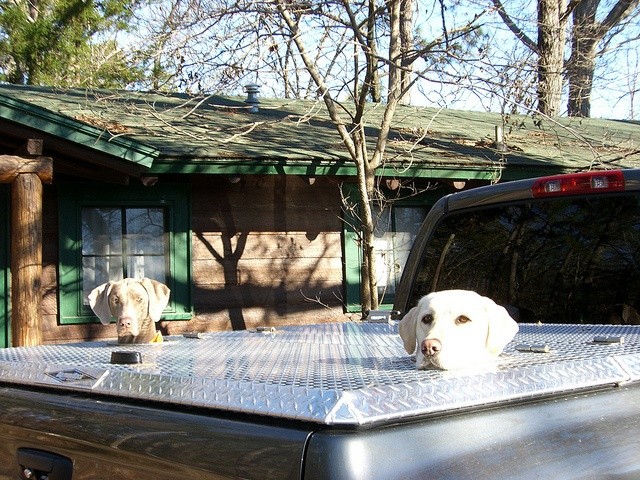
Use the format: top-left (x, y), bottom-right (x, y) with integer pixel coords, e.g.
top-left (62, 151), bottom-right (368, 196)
top-left (1, 169), bottom-right (639, 479)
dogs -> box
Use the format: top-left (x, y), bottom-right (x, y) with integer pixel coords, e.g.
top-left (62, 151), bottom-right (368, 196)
top-left (87, 276), bottom-right (171, 344)
top-left (397, 288), bottom-right (520, 373)
